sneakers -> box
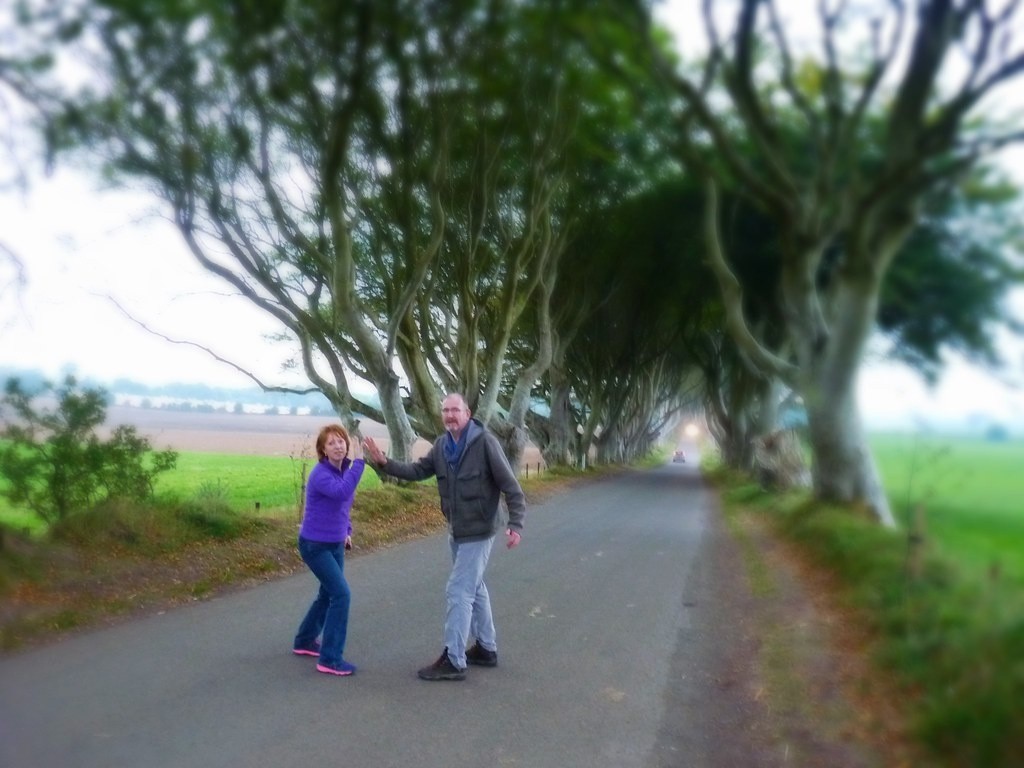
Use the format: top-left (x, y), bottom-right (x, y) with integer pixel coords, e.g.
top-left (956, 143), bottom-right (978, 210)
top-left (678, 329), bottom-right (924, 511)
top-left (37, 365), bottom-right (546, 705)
top-left (316, 654), bottom-right (354, 675)
top-left (466, 640), bottom-right (497, 665)
top-left (292, 639), bottom-right (322, 657)
top-left (418, 647), bottom-right (467, 680)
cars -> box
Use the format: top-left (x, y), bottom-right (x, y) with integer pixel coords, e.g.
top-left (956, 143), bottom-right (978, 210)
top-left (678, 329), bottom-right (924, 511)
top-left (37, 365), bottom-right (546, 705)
top-left (672, 450), bottom-right (687, 463)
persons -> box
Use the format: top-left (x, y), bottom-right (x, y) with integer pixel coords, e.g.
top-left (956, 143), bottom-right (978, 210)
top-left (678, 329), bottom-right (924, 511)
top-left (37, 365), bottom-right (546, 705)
top-left (362, 392), bottom-right (527, 680)
top-left (291, 425), bottom-right (366, 675)
